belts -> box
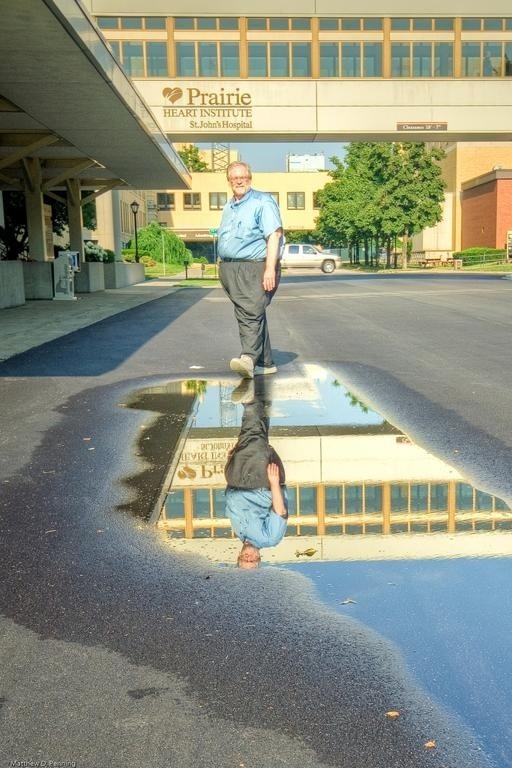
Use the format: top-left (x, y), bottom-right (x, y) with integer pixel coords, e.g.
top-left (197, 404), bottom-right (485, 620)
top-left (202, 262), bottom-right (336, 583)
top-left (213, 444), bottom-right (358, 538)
top-left (221, 257), bottom-right (266, 262)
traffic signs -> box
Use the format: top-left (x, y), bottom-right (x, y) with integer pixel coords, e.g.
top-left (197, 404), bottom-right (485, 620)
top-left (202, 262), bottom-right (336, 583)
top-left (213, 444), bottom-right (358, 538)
top-left (209, 228), bottom-right (217, 234)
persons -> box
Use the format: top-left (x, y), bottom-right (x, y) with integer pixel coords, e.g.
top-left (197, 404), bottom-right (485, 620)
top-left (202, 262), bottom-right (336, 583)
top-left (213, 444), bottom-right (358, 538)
top-left (221, 378), bottom-right (289, 568)
top-left (215, 162), bottom-right (286, 379)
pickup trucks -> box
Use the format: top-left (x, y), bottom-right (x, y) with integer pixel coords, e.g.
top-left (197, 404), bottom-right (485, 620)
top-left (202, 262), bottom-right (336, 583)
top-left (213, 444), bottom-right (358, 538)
top-left (280, 243), bottom-right (343, 274)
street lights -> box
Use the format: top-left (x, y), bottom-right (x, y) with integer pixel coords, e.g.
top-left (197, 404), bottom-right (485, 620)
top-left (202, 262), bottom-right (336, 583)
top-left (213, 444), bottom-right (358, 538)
top-left (129, 201), bottom-right (142, 264)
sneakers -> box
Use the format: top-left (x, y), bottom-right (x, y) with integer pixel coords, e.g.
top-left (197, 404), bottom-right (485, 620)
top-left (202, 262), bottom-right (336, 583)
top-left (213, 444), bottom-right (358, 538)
top-left (231, 378), bottom-right (255, 405)
top-left (230, 355), bottom-right (254, 379)
top-left (255, 366), bottom-right (277, 375)
